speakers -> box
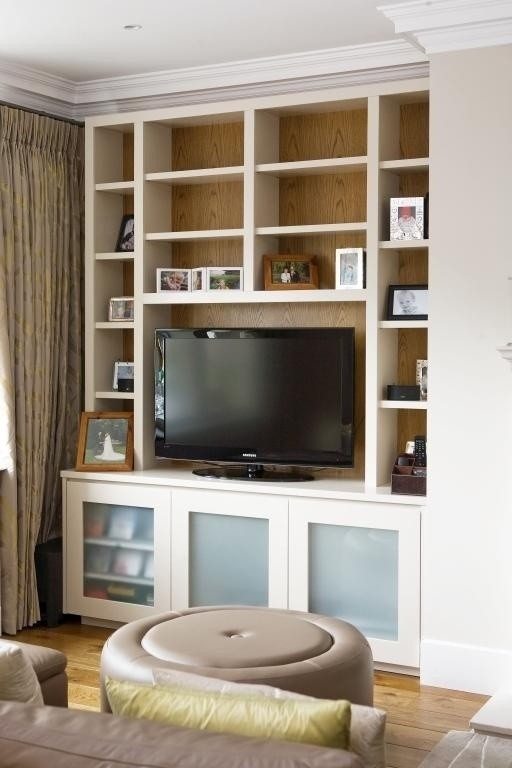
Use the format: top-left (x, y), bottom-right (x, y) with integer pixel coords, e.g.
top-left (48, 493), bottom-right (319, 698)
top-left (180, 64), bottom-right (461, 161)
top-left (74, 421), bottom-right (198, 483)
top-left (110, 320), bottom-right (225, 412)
top-left (118, 378), bottom-right (134, 392)
top-left (386, 384), bottom-right (421, 400)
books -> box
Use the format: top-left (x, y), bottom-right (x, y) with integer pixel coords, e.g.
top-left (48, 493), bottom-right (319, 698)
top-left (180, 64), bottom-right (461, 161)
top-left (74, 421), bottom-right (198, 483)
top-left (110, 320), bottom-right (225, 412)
top-left (86, 507), bottom-right (152, 603)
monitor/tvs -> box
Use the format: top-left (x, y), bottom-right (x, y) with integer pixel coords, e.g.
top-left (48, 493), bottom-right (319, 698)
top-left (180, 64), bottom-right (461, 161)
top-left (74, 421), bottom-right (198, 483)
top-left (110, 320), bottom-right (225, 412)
top-left (154, 326), bottom-right (355, 482)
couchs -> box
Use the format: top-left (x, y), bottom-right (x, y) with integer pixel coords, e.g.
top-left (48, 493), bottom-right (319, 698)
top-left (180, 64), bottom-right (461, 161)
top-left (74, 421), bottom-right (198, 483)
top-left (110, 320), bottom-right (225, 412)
top-left (96, 603), bottom-right (375, 712)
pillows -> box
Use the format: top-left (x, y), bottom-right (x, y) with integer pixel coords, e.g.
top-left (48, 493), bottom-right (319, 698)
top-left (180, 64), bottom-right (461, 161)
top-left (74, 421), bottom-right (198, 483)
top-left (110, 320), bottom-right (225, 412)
top-left (152, 667), bottom-right (387, 764)
top-left (1, 641), bottom-right (44, 702)
top-left (101, 681), bottom-right (351, 755)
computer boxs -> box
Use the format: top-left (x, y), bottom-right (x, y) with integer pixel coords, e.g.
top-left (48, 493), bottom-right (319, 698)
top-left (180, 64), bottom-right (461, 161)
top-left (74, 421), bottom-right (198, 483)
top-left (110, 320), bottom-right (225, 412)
top-left (34, 537), bottom-right (73, 628)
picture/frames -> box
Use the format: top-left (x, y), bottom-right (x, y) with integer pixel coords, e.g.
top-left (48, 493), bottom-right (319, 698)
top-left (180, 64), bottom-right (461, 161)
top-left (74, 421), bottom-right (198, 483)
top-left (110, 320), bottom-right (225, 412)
top-left (155, 248), bottom-right (365, 292)
top-left (386, 283), bottom-right (430, 321)
top-left (384, 195), bottom-right (428, 241)
top-left (110, 360), bottom-right (136, 393)
top-left (416, 357), bottom-right (431, 397)
top-left (77, 410), bottom-right (135, 471)
top-left (114, 214), bottom-right (136, 252)
top-left (108, 291), bottom-right (136, 321)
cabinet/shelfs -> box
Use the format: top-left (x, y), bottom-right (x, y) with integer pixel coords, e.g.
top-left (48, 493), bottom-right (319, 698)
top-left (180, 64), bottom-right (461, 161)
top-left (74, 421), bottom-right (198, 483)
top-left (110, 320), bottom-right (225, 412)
top-left (79, 72), bottom-right (430, 495)
top-left (180, 491), bottom-right (423, 674)
top-left (63, 471), bottom-right (175, 621)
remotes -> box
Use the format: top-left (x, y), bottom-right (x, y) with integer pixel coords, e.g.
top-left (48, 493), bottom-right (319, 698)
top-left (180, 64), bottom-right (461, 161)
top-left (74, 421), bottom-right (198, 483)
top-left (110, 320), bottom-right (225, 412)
top-left (415, 435), bottom-right (426, 467)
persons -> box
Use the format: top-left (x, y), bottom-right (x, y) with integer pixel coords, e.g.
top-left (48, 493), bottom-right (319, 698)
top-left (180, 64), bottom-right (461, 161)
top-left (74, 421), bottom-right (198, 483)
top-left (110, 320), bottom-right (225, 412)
top-left (93, 432), bottom-right (125, 460)
top-left (289, 267), bottom-right (298, 280)
top-left (398, 205), bottom-right (416, 232)
top-left (399, 293), bottom-right (416, 312)
top-left (280, 266), bottom-right (290, 283)
top-left (114, 305), bottom-right (127, 318)
top-left (344, 264), bottom-right (356, 283)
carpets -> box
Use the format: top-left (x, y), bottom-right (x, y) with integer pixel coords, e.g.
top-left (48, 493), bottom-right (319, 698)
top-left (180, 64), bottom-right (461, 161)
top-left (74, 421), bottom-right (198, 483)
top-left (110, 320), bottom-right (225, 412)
top-left (414, 728), bottom-right (512, 768)
